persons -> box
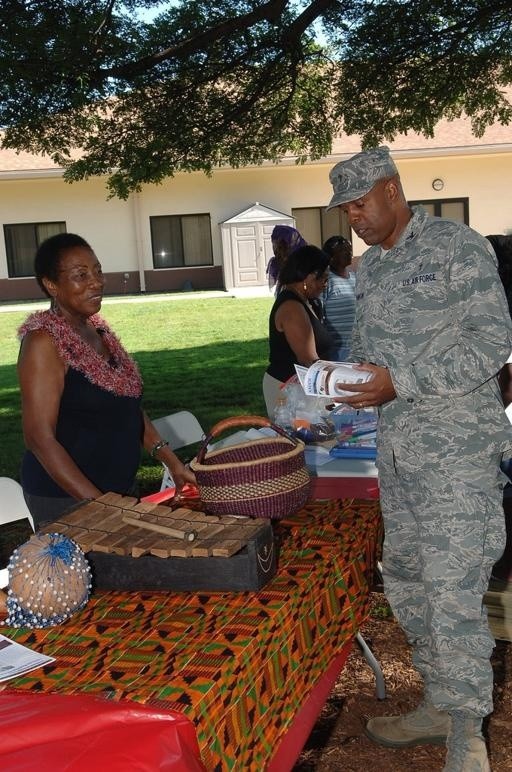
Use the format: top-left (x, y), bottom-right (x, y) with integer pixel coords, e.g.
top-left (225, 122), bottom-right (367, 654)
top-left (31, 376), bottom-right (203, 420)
top-left (325, 143), bottom-right (510, 771)
top-left (482, 232), bottom-right (511, 585)
top-left (262, 223), bottom-right (360, 427)
top-left (17, 232), bottom-right (198, 533)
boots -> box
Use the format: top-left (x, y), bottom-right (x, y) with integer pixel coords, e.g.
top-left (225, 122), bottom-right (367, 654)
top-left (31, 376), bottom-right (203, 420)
top-left (364, 696), bottom-right (450, 747)
top-left (445, 716), bottom-right (490, 772)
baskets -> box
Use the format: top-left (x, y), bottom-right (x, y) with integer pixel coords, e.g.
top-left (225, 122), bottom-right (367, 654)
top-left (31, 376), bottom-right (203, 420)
top-left (190, 413), bottom-right (311, 522)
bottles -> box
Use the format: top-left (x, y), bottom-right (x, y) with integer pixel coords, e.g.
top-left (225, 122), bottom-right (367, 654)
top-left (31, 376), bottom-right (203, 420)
top-left (274, 397), bottom-right (292, 433)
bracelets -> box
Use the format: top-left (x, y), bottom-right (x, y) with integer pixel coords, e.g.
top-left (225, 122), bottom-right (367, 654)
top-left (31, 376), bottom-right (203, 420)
top-left (150, 439), bottom-right (169, 459)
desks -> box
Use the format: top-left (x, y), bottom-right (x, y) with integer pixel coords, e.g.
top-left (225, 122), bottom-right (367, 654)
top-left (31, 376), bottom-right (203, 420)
top-left (0, 450), bottom-right (385, 772)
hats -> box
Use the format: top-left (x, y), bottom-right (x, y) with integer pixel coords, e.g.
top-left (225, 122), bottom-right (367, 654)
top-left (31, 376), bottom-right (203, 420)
top-left (324, 145), bottom-right (400, 212)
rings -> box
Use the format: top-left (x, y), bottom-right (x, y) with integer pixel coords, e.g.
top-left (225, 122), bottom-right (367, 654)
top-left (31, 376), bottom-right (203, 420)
top-left (360, 403), bottom-right (364, 408)
